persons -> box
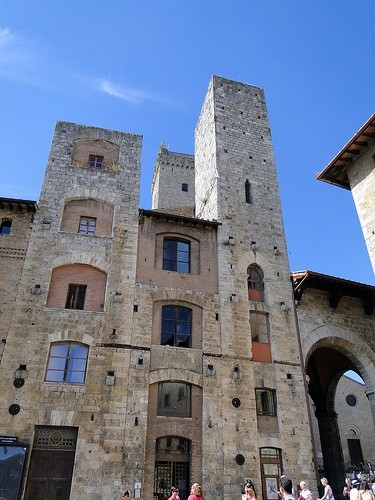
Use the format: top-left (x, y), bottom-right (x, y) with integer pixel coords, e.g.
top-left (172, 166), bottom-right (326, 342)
top-left (188, 482), bottom-right (207, 500)
top-left (278, 475), bottom-right (375, 500)
top-left (242, 483), bottom-right (261, 500)
top-left (167, 486), bottom-right (181, 500)
top-left (121, 490), bottom-right (130, 500)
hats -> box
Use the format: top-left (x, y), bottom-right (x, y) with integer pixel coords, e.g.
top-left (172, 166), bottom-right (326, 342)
top-left (351, 480), bottom-right (360, 486)
